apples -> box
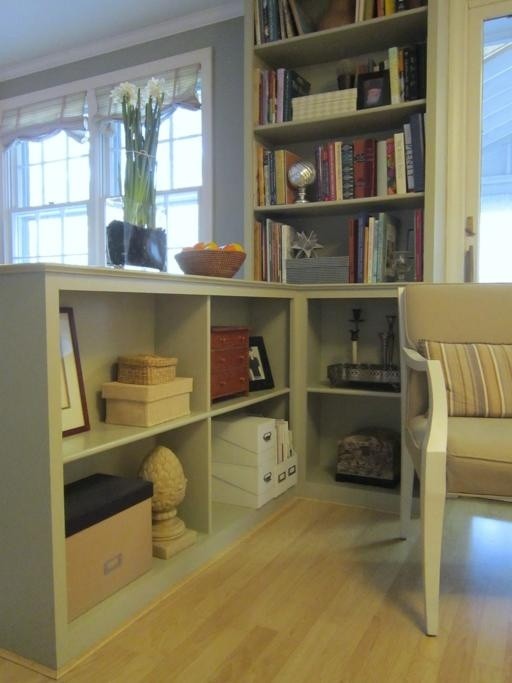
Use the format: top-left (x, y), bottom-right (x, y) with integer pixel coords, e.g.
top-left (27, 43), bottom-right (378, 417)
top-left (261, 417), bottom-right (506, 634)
top-left (183, 242), bottom-right (245, 252)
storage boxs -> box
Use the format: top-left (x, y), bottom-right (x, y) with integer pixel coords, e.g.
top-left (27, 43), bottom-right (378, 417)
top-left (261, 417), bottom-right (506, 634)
top-left (99, 374), bottom-right (193, 430)
top-left (209, 411), bottom-right (297, 511)
top-left (63, 469), bottom-right (164, 627)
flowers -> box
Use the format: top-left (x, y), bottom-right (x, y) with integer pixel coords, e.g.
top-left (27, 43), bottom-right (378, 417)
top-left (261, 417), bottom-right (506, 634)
top-left (105, 73), bottom-right (170, 232)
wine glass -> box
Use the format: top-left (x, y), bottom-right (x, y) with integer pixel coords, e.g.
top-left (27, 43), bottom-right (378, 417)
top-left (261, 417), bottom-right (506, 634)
top-left (390, 252), bottom-right (415, 284)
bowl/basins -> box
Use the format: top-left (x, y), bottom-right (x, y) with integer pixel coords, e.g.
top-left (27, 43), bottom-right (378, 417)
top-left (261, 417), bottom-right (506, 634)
top-left (173, 248), bottom-right (247, 279)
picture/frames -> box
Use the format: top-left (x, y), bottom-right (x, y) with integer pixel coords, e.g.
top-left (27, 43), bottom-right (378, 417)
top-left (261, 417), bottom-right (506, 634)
top-left (244, 333), bottom-right (275, 394)
top-left (353, 66), bottom-right (390, 110)
top-left (58, 302), bottom-right (93, 439)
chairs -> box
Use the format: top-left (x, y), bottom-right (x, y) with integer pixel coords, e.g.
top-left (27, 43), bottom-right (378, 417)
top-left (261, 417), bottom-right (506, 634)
top-left (385, 276), bottom-right (512, 638)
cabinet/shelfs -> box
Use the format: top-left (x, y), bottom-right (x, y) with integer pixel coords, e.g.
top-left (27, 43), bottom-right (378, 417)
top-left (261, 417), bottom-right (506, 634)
top-left (239, 0), bottom-right (449, 289)
top-left (1, 255), bottom-right (292, 671)
top-left (293, 293), bottom-right (421, 518)
top-left (209, 321), bottom-right (255, 407)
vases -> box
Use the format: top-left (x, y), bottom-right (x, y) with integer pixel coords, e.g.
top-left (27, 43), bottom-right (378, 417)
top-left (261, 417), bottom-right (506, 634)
top-left (101, 194), bottom-right (171, 276)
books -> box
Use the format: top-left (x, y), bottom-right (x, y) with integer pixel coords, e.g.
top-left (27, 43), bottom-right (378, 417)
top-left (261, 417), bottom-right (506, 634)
top-left (257, 146), bottom-right (306, 206)
top-left (355, 0), bottom-right (407, 23)
top-left (338, 46), bottom-right (427, 110)
top-left (256, 68), bottom-right (312, 125)
top-left (252, 0), bottom-right (313, 44)
top-left (291, 88), bottom-right (357, 122)
top-left (315, 114), bottom-right (427, 201)
top-left (286, 256), bottom-right (349, 286)
top-left (254, 217), bottom-right (298, 286)
top-left (347, 208), bottom-right (424, 283)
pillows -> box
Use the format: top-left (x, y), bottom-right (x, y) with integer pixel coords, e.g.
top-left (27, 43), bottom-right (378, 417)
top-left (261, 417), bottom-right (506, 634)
top-left (414, 332), bottom-right (512, 424)
